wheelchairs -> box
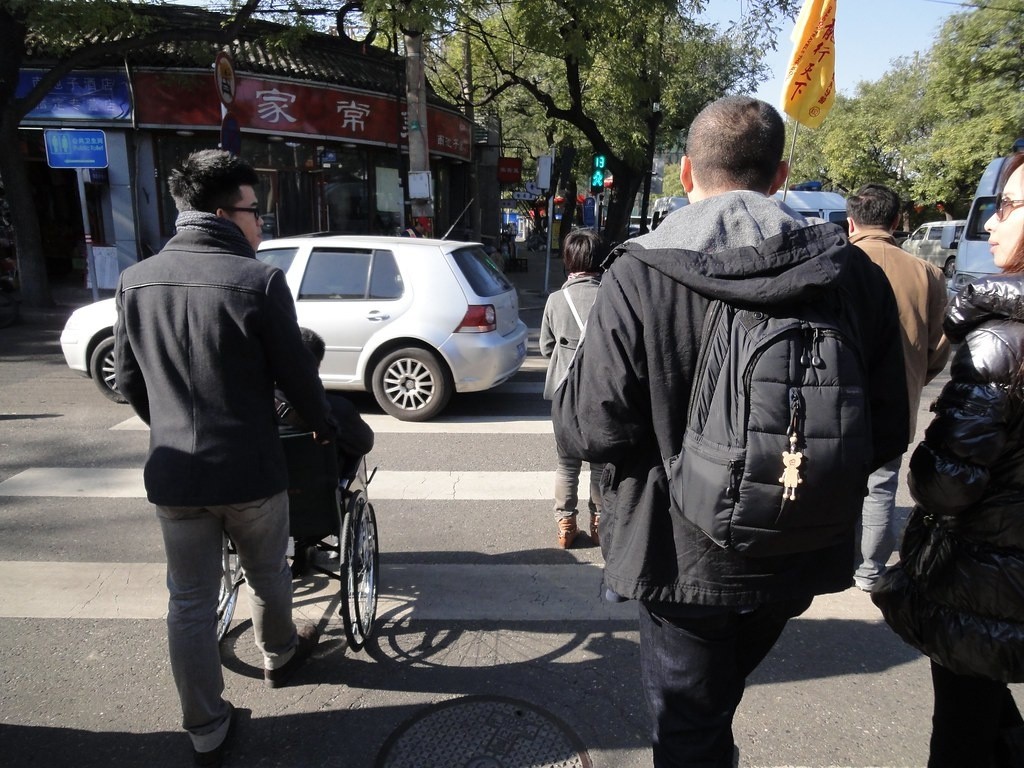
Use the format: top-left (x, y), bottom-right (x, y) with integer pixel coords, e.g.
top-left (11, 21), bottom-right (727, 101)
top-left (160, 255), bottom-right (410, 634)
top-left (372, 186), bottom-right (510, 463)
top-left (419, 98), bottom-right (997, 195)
top-left (214, 422), bottom-right (380, 651)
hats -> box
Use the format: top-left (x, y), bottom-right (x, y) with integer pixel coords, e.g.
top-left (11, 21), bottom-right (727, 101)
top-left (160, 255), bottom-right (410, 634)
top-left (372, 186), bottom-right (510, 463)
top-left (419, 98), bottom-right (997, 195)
top-left (418, 217), bottom-right (432, 233)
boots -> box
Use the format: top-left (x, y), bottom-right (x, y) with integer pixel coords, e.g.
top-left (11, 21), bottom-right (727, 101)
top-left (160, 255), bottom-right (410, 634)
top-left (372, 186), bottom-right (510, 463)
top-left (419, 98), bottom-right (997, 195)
top-left (590, 514), bottom-right (601, 545)
top-left (557, 514), bottom-right (581, 548)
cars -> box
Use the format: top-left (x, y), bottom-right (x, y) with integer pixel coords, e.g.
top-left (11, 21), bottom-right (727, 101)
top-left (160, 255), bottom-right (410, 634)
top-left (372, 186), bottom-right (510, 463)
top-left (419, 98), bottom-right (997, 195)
top-left (628, 196), bottom-right (690, 238)
top-left (60, 230), bottom-right (529, 422)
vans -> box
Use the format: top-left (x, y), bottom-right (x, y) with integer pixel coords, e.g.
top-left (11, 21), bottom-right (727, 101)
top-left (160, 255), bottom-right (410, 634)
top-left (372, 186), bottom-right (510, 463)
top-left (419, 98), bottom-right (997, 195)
top-left (899, 219), bottom-right (966, 280)
top-left (948, 138), bottom-right (1024, 309)
top-left (774, 183), bottom-right (850, 236)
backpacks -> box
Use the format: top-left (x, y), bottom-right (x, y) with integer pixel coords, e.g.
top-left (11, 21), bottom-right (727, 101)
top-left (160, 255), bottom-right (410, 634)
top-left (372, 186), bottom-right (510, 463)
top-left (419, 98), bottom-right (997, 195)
top-left (670, 299), bottom-right (870, 567)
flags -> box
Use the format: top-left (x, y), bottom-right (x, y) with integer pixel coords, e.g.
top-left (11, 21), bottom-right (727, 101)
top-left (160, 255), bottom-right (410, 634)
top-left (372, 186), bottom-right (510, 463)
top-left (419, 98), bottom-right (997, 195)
top-left (782, 1), bottom-right (838, 132)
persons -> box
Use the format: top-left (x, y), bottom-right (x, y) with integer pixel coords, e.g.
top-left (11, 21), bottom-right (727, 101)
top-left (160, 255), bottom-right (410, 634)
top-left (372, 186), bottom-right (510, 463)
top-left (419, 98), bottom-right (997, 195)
top-left (539, 228), bottom-right (615, 544)
top-left (400, 215), bottom-right (431, 237)
top-left (244, 320), bottom-right (372, 578)
top-left (821, 187), bottom-right (949, 592)
top-left (907, 152), bottom-right (1023, 768)
top-left (550, 94), bottom-right (908, 768)
top-left (112, 148), bottom-right (353, 768)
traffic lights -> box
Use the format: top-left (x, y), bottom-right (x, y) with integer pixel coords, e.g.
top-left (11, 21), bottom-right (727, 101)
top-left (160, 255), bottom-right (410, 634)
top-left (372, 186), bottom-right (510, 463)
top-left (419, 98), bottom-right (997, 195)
top-left (591, 154), bottom-right (607, 195)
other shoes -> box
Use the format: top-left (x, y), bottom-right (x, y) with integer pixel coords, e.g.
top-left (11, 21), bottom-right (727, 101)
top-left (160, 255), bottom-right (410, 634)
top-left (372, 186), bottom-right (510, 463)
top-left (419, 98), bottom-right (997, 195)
top-left (264, 624), bottom-right (320, 688)
top-left (193, 700), bottom-right (234, 768)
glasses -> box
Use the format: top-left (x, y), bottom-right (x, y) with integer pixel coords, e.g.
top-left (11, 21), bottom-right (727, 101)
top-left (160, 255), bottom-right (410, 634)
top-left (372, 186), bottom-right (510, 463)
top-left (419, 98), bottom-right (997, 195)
top-left (216, 203), bottom-right (261, 221)
top-left (995, 192), bottom-right (1024, 220)
